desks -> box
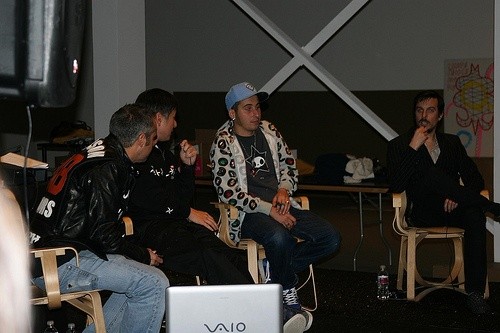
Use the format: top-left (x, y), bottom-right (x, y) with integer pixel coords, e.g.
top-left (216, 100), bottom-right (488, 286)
top-left (292, 172), bottom-right (389, 272)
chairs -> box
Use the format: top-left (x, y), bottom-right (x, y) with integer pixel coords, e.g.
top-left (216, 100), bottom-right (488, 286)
top-left (210, 196), bottom-right (318, 312)
top-left (30, 246), bottom-right (108, 333)
top-left (393, 190), bottom-right (467, 301)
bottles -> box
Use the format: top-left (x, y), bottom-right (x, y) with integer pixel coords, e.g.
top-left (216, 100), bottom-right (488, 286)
top-left (377, 264), bottom-right (389, 300)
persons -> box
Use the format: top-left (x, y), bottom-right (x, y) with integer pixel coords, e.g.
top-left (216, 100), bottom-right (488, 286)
top-left (386, 90), bottom-right (500, 315)
top-left (127, 87), bottom-right (313, 333)
top-left (29, 104), bottom-right (171, 333)
top-left (209, 82), bottom-right (342, 311)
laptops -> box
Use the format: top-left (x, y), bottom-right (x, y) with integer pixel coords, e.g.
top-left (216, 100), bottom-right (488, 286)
top-left (165, 283), bottom-right (283, 333)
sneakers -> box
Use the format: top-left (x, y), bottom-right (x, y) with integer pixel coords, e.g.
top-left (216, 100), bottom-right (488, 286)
top-left (259, 258), bottom-right (271, 284)
top-left (282, 287), bottom-right (301, 312)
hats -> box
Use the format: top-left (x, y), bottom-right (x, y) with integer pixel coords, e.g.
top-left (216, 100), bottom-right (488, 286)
top-left (225, 82), bottom-right (269, 111)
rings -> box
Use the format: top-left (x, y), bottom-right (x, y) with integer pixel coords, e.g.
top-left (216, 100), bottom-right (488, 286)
top-left (276, 202), bottom-right (282, 206)
top-left (281, 203), bottom-right (285, 206)
top-left (287, 222), bottom-right (290, 226)
top-left (285, 201), bottom-right (289, 204)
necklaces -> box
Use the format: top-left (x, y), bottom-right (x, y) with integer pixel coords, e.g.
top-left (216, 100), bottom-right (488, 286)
top-left (236, 133), bottom-right (257, 178)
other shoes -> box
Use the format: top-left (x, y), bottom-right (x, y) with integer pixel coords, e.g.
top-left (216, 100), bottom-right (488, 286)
top-left (481, 198), bottom-right (500, 222)
top-left (467, 290), bottom-right (491, 314)
top-left (283, 314), bottom-right (306, 333)
top-left (293, 310), bottom-right (313, 331)
